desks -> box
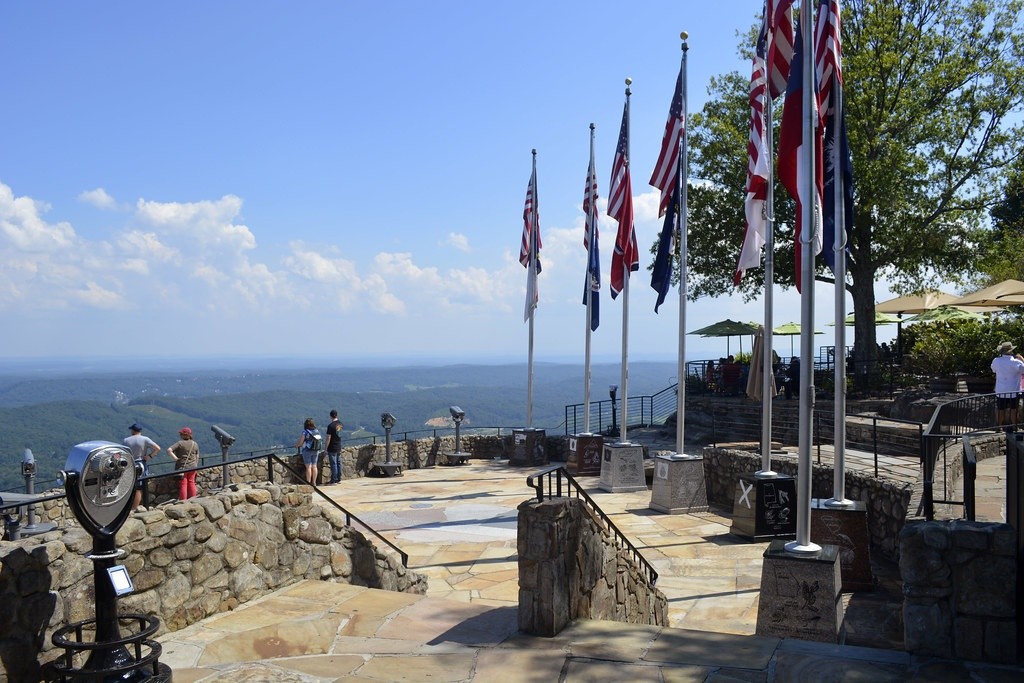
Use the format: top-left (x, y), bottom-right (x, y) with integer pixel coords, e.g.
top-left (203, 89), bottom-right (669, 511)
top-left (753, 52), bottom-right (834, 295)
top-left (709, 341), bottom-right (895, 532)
top-left (774, 376), bottom-right (796, 400)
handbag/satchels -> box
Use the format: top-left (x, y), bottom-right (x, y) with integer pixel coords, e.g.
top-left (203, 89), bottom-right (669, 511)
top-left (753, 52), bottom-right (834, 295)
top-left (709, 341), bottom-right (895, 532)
top-left (173, 473), bottom-right (184, 481)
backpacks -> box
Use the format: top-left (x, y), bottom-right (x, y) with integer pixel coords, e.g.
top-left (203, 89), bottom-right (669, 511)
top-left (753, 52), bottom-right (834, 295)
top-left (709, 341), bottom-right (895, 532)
top-left (306, 429), bottom-right (322, 451)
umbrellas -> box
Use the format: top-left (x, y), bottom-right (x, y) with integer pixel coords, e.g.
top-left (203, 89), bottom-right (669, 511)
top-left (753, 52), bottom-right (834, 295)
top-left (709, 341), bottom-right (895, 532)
top-left (685, 318), bottom-right (755, 357)
top-left (901, 305), bottom-right (989, 323)
top-left (772, 322), bottom-right (825, 357)
top-left (948, 279), bottom-right (1024, 305)
top-left (875, 290), bottom-right (1007, 365)
top-left (737, 322), bottom-right (762, 360)
top-left (828, 312), bottom-right (902, 326)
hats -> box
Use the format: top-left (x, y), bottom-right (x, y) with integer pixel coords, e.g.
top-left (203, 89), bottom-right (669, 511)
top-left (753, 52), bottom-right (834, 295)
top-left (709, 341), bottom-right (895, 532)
top-left (179, 428), bottom-right (192, 435)
top-left (997, 342), bottom-right (1017, 354)
top-left (128, 424), bottom-right (143, 432)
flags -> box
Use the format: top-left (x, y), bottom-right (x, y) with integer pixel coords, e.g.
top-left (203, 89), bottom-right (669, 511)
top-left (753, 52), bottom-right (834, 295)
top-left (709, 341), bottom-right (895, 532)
top-left (779, 0), bottom-right (822, 294)
top-left (734, 0), bottom-right (793, 287)
top-left (813, 0), bottom-right (852, 277)
top-left (607, 103), bottom-right (639, 301)
top-left (583, 160), bottom-right (602, 331)
top-left (649, 61), bottom-right (682, 314)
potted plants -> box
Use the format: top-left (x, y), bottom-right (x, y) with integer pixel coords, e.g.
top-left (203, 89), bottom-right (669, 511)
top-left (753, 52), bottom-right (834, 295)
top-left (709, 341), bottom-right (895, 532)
top-left (910, 332), bottom-right (1017, 393)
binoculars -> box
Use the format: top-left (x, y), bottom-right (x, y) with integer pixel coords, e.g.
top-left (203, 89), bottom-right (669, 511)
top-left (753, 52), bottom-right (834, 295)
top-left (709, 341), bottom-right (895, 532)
top-left (380, 412), bottom-right (397, 464)
top-left (450, 406), bottom-right (466, 454)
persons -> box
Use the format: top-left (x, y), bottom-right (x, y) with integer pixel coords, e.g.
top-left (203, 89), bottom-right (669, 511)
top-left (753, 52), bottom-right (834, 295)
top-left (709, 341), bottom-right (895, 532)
top-left (786, 356), bottom-right (800, 400)
top-left (991, 342), bottom-right (1024, 431)
top-left (325, 410), bottom-right (343, 486)
top-left (296, 418), bottom-right (319, 486)
top-left (707, 355), bottom-right (742, 391)
top-left (519, 169), bottom-right (542, 323)
top-left (124, 423), bottom-right (160, 510)
top-left (873, 341), bottom-right (895, 356)
top-left (167, 427), bottom-right (199, 500)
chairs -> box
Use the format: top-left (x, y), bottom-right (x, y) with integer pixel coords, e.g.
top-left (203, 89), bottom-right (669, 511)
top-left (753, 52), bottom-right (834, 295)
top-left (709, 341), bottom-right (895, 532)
top-left (695, 367), bottom-right (715, 394)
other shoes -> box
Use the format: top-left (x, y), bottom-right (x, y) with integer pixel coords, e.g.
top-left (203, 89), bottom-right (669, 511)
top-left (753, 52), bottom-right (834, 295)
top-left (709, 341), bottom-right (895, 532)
top-left (338, 480), bottom-right (341, 484)
top-left (326, 480), bottom-right (337, 485)
top-left (1013, 428), bottom-right (1019, 432)
top-left (996, 428), bottom-right (1003, 432)
